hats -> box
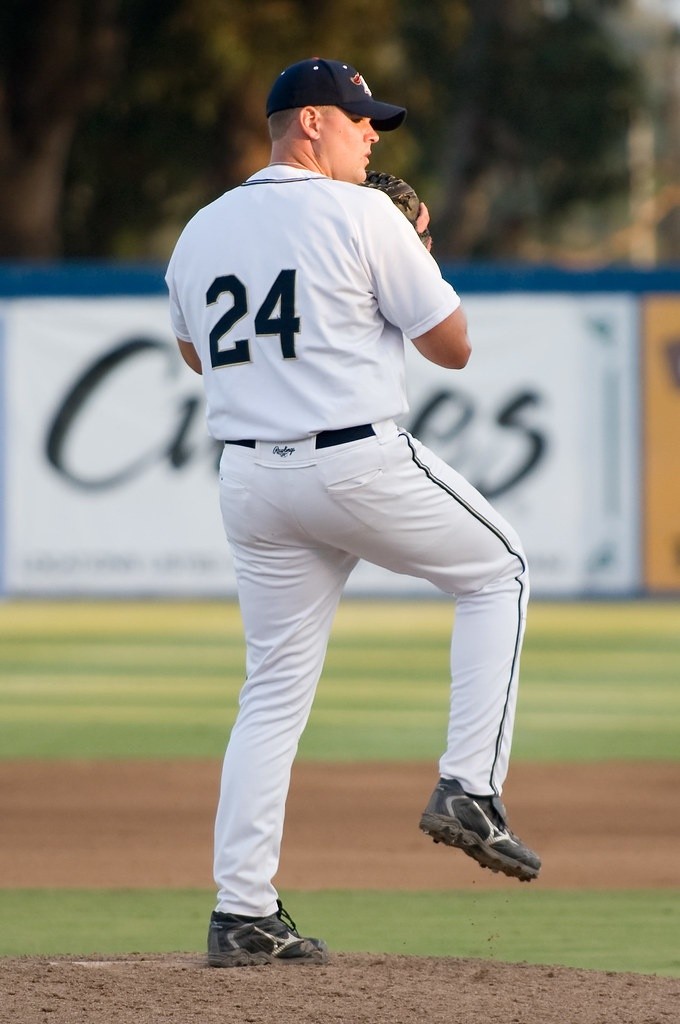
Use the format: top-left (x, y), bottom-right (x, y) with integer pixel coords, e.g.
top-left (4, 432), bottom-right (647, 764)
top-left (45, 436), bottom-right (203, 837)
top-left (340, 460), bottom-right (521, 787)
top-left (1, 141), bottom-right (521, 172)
top-left (264, 56), bottom-right (408, 134)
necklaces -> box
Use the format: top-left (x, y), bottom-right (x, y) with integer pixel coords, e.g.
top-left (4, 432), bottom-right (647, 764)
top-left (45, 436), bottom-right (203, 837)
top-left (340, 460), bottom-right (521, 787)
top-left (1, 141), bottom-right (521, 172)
top-left (267, 162), bottom-right (308, 168)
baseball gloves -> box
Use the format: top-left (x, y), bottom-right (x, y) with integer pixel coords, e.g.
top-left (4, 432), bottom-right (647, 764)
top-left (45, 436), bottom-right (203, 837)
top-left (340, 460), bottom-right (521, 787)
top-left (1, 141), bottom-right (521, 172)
top-left (359, 170), bottom-right (432, 252)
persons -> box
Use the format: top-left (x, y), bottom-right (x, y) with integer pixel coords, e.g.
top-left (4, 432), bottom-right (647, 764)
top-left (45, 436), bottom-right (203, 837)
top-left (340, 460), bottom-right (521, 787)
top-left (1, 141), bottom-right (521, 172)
top-left (164, 58), bottom-right (544, 968)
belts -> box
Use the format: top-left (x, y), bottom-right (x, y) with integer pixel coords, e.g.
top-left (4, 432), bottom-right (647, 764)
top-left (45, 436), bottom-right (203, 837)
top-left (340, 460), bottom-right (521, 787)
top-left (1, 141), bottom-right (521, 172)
top-left (227, 424), bottom-right (376, 449)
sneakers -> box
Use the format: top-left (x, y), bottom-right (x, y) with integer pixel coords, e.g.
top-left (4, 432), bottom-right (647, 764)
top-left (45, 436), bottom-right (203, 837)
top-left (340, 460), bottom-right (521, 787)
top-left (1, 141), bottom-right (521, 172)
top-left (206, 899), bottom-right (325, 966)
top-left (419, 779), bottom-right (547, 882)
